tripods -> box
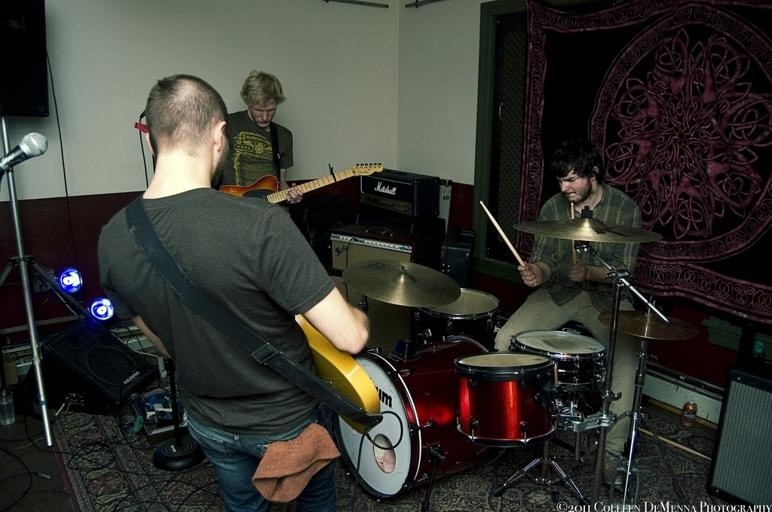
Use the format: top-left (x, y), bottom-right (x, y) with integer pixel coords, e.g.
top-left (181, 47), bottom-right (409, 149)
top-left (493, 436), bottom-right (591, 512)
top-left (0, 118), bottom-right (90, 446)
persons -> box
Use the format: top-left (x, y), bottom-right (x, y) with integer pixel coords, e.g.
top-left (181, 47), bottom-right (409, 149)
top-left (492, 138), bottom-right (642, 487)
top-left (95, 73), bottom-right (374, 511)
top-left (217, 70), bottom-right (310, 242)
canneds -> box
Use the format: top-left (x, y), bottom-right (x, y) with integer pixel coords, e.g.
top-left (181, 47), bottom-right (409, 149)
top-left (680, 402), bottom-right (697, 426)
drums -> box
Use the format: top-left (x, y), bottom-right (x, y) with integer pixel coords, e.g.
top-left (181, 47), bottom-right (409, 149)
top-left (512, 329), bottom-right (606, 385)
top-left (454, 353), bottom-right (556, 448)
top-left (412, 287), bottom-right (499, 352)
top-left (334, 334), bottom-right (505, 500)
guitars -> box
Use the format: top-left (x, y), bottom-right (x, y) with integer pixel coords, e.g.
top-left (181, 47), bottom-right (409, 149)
top-left (218, 163), bottom-right (383, 205)
top-left (294, 313), bottom-right (379, 434)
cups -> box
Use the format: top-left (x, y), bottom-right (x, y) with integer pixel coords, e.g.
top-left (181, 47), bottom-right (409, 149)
top-left (0, 390), bottom-right (17, 426)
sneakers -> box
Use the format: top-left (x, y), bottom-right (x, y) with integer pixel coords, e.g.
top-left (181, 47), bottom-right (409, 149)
top-left (604, 453), bottom-right (626, 486)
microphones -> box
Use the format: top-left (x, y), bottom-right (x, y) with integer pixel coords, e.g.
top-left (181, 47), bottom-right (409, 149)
top-left (0, 132), bottom-right (47, 171)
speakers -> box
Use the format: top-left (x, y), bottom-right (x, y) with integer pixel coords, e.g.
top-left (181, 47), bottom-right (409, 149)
top-left (41, 316), bottom-right (156, 415)
top-left (0, 0), bottom-right (49, 118)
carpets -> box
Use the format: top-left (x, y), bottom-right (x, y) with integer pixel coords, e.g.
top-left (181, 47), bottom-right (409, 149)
top-left (43, 342), bottom-right (755, 511)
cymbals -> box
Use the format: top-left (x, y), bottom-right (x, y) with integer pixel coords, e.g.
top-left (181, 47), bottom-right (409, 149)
top-left (512, 218), bottom-right (662, 244)
top-left (598, 310), bottom-right (700, 341)
top-left (343, 259), bottom-right (461, 307)
